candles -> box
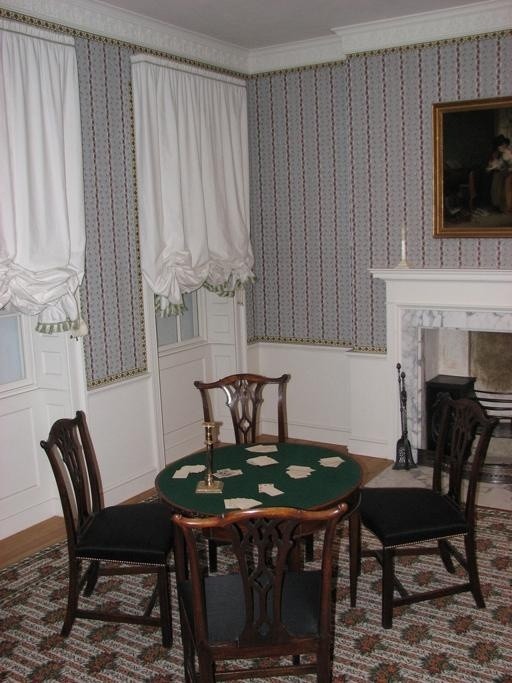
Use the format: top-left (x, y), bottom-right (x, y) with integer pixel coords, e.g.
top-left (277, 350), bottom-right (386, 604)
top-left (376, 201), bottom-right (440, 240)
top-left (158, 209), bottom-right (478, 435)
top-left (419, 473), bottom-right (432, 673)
top-left (399, 227), bottom-right (405, 258)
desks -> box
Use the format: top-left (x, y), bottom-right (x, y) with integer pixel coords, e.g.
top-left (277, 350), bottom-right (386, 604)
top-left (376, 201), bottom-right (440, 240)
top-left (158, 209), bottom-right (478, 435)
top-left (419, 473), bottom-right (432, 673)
top-left (155, 442), bottom-right (364, 607)
top-left (349, 393), bottom-right (500, 629)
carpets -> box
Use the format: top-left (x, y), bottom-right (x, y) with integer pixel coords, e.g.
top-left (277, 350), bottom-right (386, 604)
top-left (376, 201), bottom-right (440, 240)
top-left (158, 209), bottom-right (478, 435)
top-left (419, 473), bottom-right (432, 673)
top-left (0, 493), bottom-right (512, 683)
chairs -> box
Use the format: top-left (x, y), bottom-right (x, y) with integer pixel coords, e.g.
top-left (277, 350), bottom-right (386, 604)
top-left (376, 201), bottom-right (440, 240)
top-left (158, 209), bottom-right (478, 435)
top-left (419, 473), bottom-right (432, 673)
top-left (171, 502), bottom-right (348, 683)
top-left (193, 373), bottom-right (313, 573)
top-left (39, 410), bottom-right (174, 649)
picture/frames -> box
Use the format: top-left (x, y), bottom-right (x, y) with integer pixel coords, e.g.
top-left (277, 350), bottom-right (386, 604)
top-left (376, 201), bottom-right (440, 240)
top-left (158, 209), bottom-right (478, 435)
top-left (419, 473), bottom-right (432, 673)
top-left (431, 95), bottom-right (512, 238)
